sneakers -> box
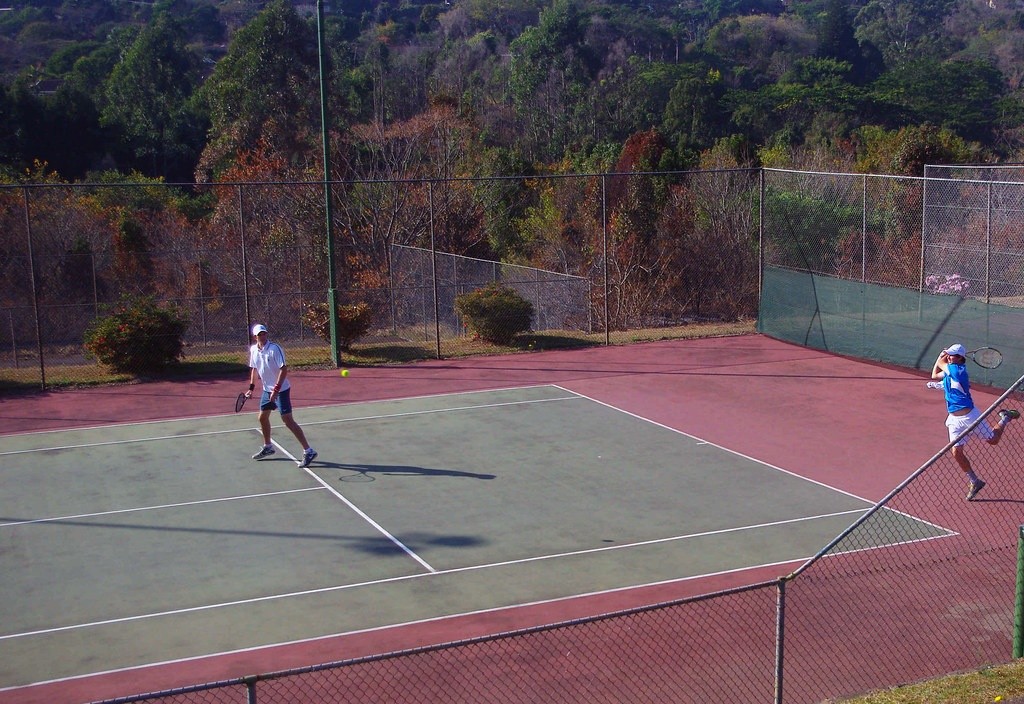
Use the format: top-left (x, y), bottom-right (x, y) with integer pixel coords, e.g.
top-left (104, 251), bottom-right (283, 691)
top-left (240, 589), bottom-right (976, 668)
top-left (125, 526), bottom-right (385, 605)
top-left (297, 449), bottom-right (318, 468)
top-left (965, 479), bottom-right (986, 500)
top-left (997, 409), bottom-right (1021, 419)
top-left (252, 446), bottom-right (275, 459)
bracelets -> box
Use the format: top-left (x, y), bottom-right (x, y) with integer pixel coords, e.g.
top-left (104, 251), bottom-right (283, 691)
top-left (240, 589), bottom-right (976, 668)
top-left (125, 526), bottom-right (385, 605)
top-left (248, 384), bottom-right (255, 391)
top-left (274, 384), bottom-right (281, 393)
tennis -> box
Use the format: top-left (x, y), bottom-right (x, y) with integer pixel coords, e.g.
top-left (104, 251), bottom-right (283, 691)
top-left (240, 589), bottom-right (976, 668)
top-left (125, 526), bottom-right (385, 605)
top-left (341, 369), bottom-right (349, 377)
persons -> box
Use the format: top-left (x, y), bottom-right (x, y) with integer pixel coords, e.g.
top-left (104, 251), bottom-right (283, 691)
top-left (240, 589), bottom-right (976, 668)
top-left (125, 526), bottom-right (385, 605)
top-left (244, 323), bottom-right (319, 468)
top-left (929, 343), bottom-right (1021, 502)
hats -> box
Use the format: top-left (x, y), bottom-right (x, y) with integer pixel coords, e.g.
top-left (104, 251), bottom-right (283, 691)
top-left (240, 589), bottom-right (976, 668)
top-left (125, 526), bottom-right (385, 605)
top-left (942, 343), bottom-right (967, 358)
top-left (253, 324), bottom-right (268, 336)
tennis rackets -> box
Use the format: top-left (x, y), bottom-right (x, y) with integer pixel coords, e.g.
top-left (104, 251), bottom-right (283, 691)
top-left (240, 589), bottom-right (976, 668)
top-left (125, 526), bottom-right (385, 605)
top-left (235, 391), bottom-right (251, 413)
top-left (966, 346), bottom-right (1004, 370)
